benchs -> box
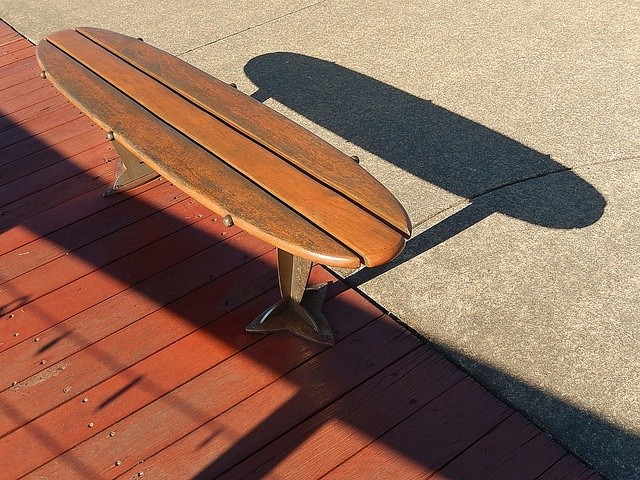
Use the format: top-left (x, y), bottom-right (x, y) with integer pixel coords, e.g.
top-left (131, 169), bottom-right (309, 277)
top-left (35, 27), bottom-right (412, 345)
